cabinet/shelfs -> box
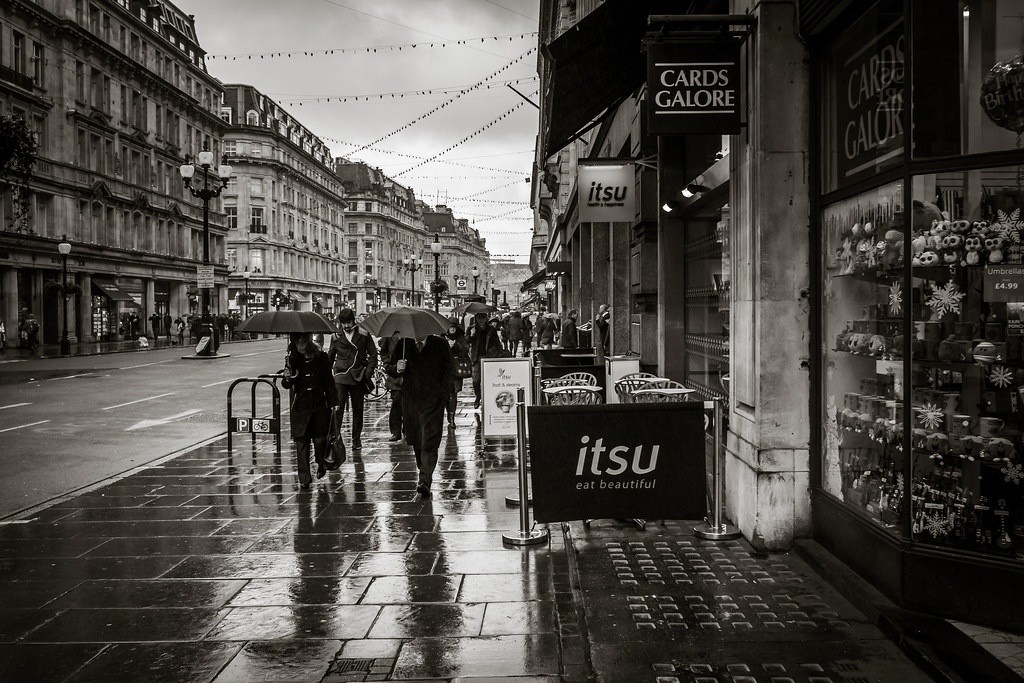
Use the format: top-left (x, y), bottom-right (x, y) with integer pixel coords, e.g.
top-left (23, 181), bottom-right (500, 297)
top-left (830, 261), bottom-right (1024, 481)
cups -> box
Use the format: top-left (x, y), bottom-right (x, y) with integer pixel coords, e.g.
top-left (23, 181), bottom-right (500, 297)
top-left (842, 302), bottom-right (1024, 439)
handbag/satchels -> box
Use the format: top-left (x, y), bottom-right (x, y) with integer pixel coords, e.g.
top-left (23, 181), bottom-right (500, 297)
top-left (323, 407), bottom-right (345, 470)
top-left (454, 354), bottom-right (473, 379)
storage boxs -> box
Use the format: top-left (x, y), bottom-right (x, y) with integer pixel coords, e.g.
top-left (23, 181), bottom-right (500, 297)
top-left (847, 488), bottom-right (867, 507)
top-left (882, 508), bottom-right (902, 527)
top-left (869, 501), bottom-right (883, 519)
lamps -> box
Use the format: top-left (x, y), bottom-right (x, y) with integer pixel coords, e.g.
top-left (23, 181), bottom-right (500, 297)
top-left (662, 200), bottom-right (679, 213)
top-left (681, 184), bottom-right (697, 198)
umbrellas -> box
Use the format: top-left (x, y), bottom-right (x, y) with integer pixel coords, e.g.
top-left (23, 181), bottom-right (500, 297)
top-left (355, 306), bottom-right (453, 374)
top-left (449, 302), bottom-right (499, 332)
top-left (234, 310), bottom-right (342, 378)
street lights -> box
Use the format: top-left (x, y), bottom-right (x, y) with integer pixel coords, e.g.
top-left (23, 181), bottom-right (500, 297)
top-left (57, 234), bottom-right (72, 354)
top-left (471, 266), bottom-right (480, 293)
top-left (242, 265), bottom-right (250, 319)
top-left (178, 139), bottom-right (233, 356)
top-left (403, 250), bottom-right (423, 306)
top-left (429, 233), bottom-right (442, 313)
top-left (373, 289), bottom-right (378, 313)
top-left (338, 281), bottom-right (342, 313)
top-left (490, 280), bottom-right (495, 316)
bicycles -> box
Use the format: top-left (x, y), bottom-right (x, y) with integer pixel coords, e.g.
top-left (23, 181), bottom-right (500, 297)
top-left (363, 347), bottom-right (391, 401)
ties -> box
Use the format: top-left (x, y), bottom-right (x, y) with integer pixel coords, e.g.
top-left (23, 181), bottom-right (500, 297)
top-left (416, 341), bottom-right (423, 354)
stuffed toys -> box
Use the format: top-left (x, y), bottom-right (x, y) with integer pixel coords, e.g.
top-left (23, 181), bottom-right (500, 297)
top-left (835, 199), bottom-right (1024, 462)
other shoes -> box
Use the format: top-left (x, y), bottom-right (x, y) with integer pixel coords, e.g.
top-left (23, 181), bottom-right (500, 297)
top-left (353, 440), bottom-right (361, 450)
top-left (417, 483), bottom-right (430, 497)
top-left (317, 464), bottom-right (325, 479)
top-left (474, 397), bottom-right (481, 409)
top-left (447, 411), bottom-right (456, 428)
top-left (300, 482), bottom-right (307, 489)
top-left (388, 433), bottom-right (400, 442)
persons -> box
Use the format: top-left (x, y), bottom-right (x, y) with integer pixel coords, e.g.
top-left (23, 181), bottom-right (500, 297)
top-left (121, 307), bottom-right (555, 441)
top-left (282, 332), bottom-right (338, 487)
top-left (596, 304), bottom-right (610, 356)
top-left (563, 309), bottom-right (579, 349)
top-left (23, 314), bottom-right (40, 354)
top-left (328, 308), bottom-right (378, 450)
top-left (465, 313), bottom-right (502, 409)
top-left (385, 335), bottom-right (453, 496)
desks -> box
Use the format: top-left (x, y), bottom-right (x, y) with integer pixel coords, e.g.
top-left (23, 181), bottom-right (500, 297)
top-left (614, 377), bottom-right (671, 384)
top-left (541, 379), bottom-right (588, 385)
top-left (631, 388), bottom-right (697, 402)
top-left (542, 385), bottom-right (603, 403)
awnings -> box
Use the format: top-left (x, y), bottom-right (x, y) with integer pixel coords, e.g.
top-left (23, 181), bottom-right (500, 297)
top-left (536, 0), bottom-right (729, 169)
top-left (314, 293), bottom-right (329, 310)
top-left (519, 268), bottom-right (546, 307)
top-left (289, 291), bottom-right (309, 302)
top-left (92, 275), bottom-right (135, 301)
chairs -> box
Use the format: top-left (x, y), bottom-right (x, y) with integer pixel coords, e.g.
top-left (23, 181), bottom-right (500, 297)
top-left (540, 372), bottom-right (690, 406)
